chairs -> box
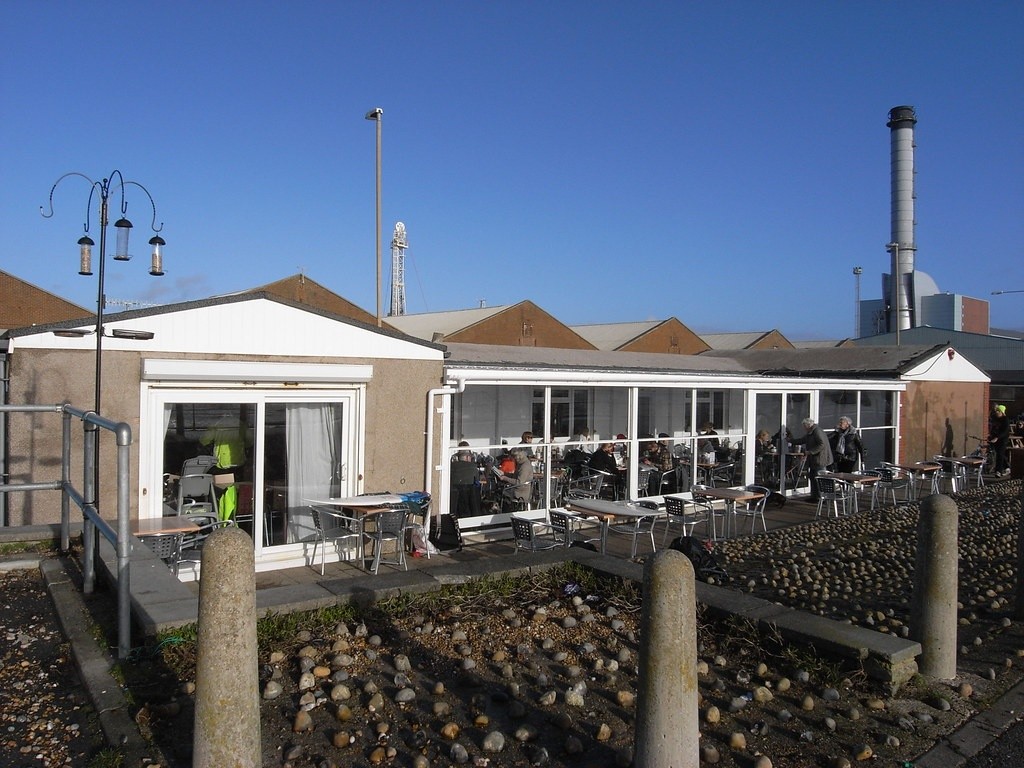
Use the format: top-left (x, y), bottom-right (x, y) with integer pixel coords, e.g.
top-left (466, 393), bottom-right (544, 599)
top-left (661, 496), bottom-right (710, 547)
top-left (690, 485), bottom-right (727, 539)
top-left (135, 532), bottom-right (185, 578)
top-left (604, 499), bottom-right (659, 558)
top-left (308, 505), bottom-right (364, 576)
top-left (726, 485), bottom-right (771, 535)
top-left (398, 497), bottom-right (432, 560)
top-left (813, 475), bottom-right (855, 520)
top-left (847, 470), bottom-right (882, 509)
top-left (509, 515), bottom-right (568, 555)
top-left (264, 479), bottom-right (285, 544)
top-left (873, 455), bottom-right (987, 507)
top-left (229, 482), bottom-right (269, 548)
top-left (549, 509), bottom-right (603, 553)
top-left (448, 454), bottom-right (809, 516)
top-left (177, 474), bottom-right (220, 549)
top-left (817, 470), bottom-right (853, 516)
top-left (355, 509), bottom-right (409, 575)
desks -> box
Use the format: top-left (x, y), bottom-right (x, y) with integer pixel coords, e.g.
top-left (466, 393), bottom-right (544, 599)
top-left (215, 483), bottom-right (270, 489)
top-left (765, 452), bottom-right (804, 483)
top-left (825, 472), bottom-right (881, 513)
top-left (533, 470), bottom-right (566, 505)
top-left (690, 488), bottom-right (765, 540)
top-left (681, 460), bottom-right (720, 488)
top-left (938, 457), bottom-right (986, 491)
top-left (568, 499), bottom-right (667, 555)
top-left (130, 516), bottom-right (201, 578)
top-left (615, 462), bottom-right (655, 499)
top-left (299, 491), bottom-right (427, 569)
top-left (890, 463), bottom-right (942, 502)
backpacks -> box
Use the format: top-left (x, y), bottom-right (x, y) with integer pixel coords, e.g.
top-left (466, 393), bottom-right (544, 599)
top-left (427, 512), bottom-right (463, 553)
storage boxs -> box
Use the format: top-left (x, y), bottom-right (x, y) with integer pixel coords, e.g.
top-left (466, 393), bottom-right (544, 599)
top-left (212, 472), bottom-right (234, 485)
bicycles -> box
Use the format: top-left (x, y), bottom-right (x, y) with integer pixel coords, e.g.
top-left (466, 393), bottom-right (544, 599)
top-left (970, 435), bottom-right (997, 475)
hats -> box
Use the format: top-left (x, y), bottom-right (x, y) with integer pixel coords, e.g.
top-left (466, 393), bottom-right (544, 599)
top-left (997, 405), bottom-right (1007, 416)
top-left (617, 434), bottom-right (627, 439)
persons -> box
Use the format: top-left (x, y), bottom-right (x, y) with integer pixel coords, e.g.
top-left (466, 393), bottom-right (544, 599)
top-left (450, 432), bottom-right (562, 518)
top-left (830, 415), bottom-right (867, 487)
top-left (987, 403), bottom-right (1024, 478)
top-left (572, 426), bottom-right (628, 502)
top-left (639, 433), bottom-right (688, 498)
top-left (696, 422), bottom-right (719, 486)
top-left (786, 417), bottom-right (833, 505)
top-left (199, 403), bottom-right (254, 482)
top-left (756, 428), bottom-right (776, 487)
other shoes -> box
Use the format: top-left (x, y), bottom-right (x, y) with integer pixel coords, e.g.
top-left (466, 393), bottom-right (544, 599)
top-left (995, 471), bottom-right (1002, 478)
top-left (1001, 467), bottom-right (1011, 476)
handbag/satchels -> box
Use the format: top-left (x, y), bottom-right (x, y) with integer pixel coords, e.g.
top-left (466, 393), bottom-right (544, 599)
top-left (667, 535), bottom-right (728, 584)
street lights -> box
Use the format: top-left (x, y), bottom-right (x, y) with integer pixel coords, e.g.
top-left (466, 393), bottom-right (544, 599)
top-left (40, 168), bottom-right (167, 581)
top-left (886, 243), bottom-right (899, 345)
top-left (365, 107), bottom-right (383, 327)
